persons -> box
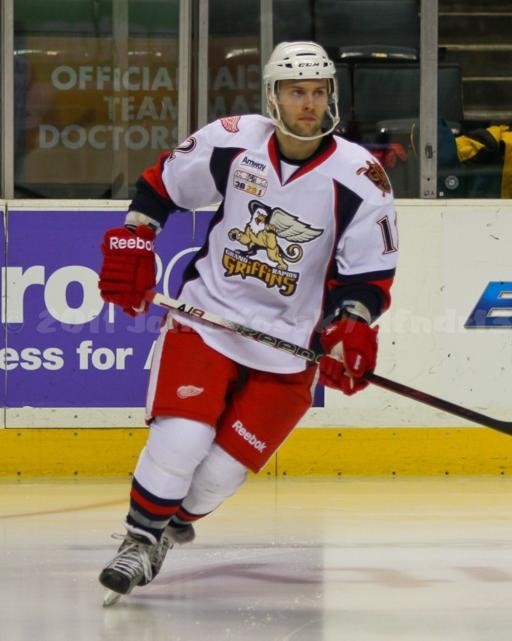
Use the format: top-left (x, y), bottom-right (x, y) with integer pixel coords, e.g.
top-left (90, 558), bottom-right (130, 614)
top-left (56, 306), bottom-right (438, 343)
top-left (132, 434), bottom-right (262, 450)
top-left (99, 41), bottom-right (400, 595)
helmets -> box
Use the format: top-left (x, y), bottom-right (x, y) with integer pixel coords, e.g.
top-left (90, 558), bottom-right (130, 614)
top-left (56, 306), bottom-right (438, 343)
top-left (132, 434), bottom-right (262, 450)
top-left (263, 41), bottom-right (340, 141)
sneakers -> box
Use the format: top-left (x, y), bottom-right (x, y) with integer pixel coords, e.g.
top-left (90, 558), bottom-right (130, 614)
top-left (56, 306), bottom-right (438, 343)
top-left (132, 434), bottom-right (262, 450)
top-left (101, 528), bottom-right (195, 593)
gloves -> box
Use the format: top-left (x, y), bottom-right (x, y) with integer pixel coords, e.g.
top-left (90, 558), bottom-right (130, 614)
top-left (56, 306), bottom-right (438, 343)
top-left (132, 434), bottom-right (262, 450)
top-left (318, 321), bottom-right (378, 397)
top-left (98, 225), bottom-right (157, 318)
